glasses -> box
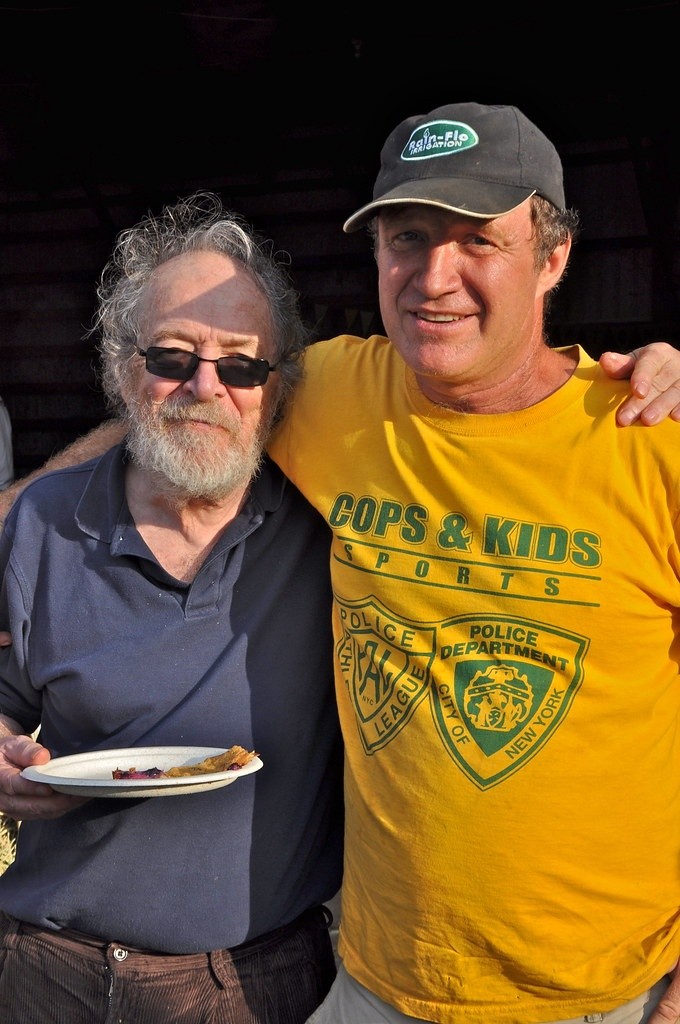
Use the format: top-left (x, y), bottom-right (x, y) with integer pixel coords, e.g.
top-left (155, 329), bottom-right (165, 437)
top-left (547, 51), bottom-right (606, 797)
top-left (139, 346), bottom-right (278, 387)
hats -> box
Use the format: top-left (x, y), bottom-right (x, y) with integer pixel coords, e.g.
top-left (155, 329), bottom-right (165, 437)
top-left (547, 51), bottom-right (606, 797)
top-left (342, 101), bottom-right (567, 235)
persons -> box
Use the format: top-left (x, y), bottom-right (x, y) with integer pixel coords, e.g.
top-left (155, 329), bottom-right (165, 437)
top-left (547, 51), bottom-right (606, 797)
top-left (0, 199), bottom-right (680, 1023)
top-left (2, 102), bottom-right (680, 1024)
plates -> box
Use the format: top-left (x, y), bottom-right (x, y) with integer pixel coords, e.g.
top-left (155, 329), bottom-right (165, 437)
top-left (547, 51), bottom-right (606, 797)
top-left (19, 746), bottom-right (263, 797)
